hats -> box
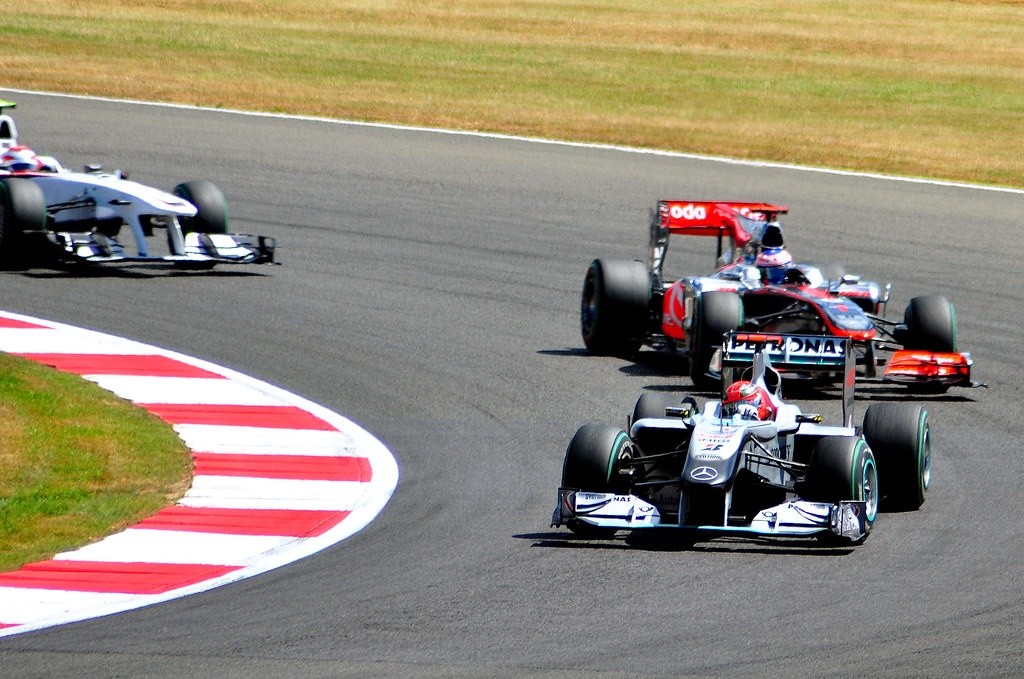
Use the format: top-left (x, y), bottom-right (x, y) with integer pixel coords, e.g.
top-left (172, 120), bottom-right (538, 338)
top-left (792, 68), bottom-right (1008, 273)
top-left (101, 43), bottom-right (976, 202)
top-left (722, 381), bottom-right (768, 423)
top-left (2, 145), bottom-right (41, 173)
top-left (754, 248), bottom-right (793, 287)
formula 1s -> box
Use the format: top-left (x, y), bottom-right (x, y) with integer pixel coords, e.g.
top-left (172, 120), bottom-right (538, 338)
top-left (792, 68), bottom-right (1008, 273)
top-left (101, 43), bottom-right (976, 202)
top-left (0, 99), bottom-right (282, 280)
top-left (580, 197), bottom-right (990, 398)
top-left (550, 329), bottom-right (935, 556)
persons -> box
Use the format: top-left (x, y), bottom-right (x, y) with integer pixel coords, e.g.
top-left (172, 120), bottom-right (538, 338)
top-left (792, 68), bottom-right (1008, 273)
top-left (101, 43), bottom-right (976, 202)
top-left (754, 248), bottom-right (795, 284)
top-left (2, 145), bottom-right (43, 173)
top-left (722, 380), bottom-right (776, 421)
